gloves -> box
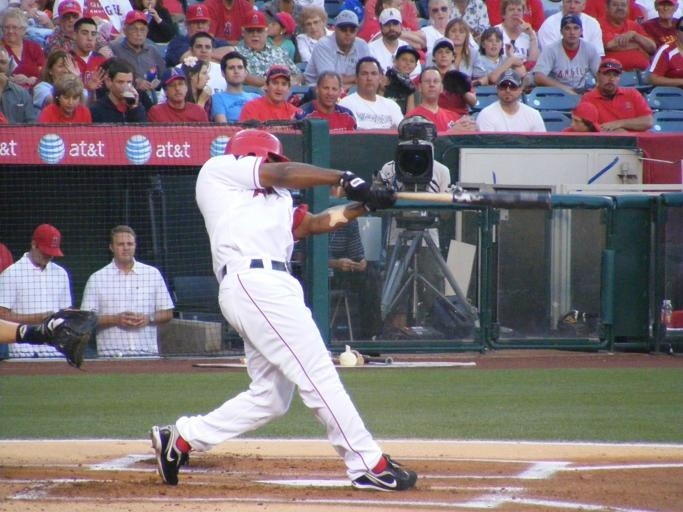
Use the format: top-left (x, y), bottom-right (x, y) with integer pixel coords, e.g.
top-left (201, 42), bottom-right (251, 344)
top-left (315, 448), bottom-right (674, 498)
top-left (362, 184), bottom-right (395, 211)
top-left (338, 169), bottom-right (371, 201)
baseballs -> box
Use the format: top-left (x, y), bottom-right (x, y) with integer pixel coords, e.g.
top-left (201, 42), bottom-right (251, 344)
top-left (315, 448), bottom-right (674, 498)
top-left (340, 353), bottom-right (356, 365)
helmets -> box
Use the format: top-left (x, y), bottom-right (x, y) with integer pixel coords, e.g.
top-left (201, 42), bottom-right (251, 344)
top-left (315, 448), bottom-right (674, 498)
top-left (224, 128), bottom-right (291, 162)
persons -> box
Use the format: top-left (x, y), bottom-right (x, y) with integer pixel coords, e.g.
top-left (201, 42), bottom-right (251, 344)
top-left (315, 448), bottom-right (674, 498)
top-left (147, 127), bottom-right (418, 492)
top-left (371, 149), bottom-right (453, 329)
top-left (1, 222), bottom-right (176, 360)
top-left (1, 0), bottom-right (682, 131)
top-left (290, 181), bottom-right (392, 341)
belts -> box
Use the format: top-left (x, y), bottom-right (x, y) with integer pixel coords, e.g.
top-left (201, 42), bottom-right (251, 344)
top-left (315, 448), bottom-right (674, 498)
top-left (222, 259), bottom-right (293, 278)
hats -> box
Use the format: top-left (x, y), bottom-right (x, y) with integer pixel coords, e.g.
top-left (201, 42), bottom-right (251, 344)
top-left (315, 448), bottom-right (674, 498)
top-left (267, 65), bottom-right (290, 81)
top-left (396, 45), bottom-right (420, 60)
top-left (599, 58), bottom-right (623, 74)
top-left (656, 0), bottom-right (677, 5)
top-left (124, 10), bottom-right (148, 25)
top-left (561, 14), bottom-right (583, 37)
top-left (335, 10), bottom-right (360, 27)
top-left (267, 9), bottom-right (296, 34)
top-left (32, 224), bottom-right (64, 256)
top-left (244, 11), bottom-right (267, 29)
top-left (496, 68), bottom-right (521, 88)
top-left (433, 38), bottom-right (455, 50)
top-left (161, 67), bottom-right (186, 85)
top-left (58, 0), bottom-right (81, 16)
top-left (185, 4), bottom-right (210, 21)
top-left (379, 8), bottom-right (403, 25)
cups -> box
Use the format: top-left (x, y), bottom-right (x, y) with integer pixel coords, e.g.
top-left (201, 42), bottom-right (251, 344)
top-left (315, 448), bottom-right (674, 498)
top-left (143, 10), bottom-right (152, 24)
top-left (121, 84), bottom-right (136, 106)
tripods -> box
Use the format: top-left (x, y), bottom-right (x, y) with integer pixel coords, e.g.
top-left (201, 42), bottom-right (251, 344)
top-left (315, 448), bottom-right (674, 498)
top-left (372, 184), bottom-right (479, 341)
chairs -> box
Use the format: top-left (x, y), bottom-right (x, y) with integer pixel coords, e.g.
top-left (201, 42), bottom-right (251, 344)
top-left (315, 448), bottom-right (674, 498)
top-left (170, 274), bottom-right (243, 349)
top-left (291, 258), bottom-right (352, 340)
top-left (0, 0), bottom-right (683, 134)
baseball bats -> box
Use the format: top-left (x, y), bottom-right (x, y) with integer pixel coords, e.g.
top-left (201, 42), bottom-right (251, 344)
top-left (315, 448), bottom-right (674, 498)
top-left (394, 191), bottom-right (552, 211)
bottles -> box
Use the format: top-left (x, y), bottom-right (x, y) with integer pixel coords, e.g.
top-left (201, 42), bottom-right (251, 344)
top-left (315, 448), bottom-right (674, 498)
top-left (661, 300), bottom-right (673, 328)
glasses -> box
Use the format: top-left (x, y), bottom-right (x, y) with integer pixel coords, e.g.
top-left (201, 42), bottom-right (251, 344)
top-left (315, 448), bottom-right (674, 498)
top-left (340, 26), bottom-right (356, 33)
top-left (498, 80), bottom-right (518, 91)
top-left (600, 63), bottom-right (622, 69)
top-left (268, 69), bottom-right (290, 78)
top-left (432, 7), bottom-right (447, 13)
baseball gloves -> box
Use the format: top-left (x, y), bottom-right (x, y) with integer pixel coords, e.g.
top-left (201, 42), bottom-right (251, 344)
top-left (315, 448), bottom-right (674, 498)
top-left (41, 311), bottom-right (97, 368)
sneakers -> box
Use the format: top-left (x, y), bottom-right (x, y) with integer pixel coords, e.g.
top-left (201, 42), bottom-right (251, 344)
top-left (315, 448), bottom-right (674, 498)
top-left (352, 454), bottom-right (417, 491)
top-left (149, 424), bottom-right (189, 484)
